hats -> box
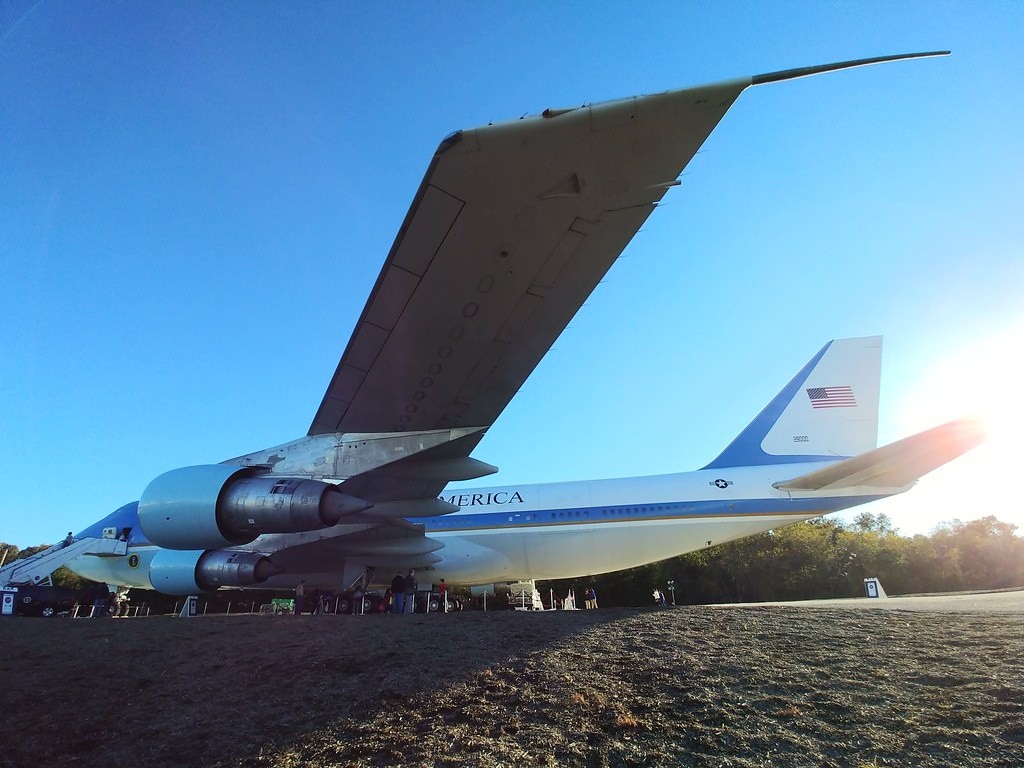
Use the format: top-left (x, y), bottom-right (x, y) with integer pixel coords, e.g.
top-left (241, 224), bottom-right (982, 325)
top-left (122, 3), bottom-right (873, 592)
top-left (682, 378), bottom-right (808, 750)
top-left (408, 569), bottom-right (416, 573)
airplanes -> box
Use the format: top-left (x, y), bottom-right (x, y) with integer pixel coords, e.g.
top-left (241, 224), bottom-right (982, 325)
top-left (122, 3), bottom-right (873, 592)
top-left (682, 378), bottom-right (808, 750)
top-left (0, 50), bottom-right (987, 610)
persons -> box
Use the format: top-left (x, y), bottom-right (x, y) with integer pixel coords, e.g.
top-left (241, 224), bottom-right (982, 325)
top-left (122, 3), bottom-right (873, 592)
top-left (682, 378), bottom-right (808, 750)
top-left (584, 587), bottom-right (598, 609)
top-left (381, 588), bottom-right (393, 614)
top-left (62, 531), bottom-right (72, 547)
top-left (438, 578), bottom-right (448, 612)
top-left (404, 568), bottom-right (417, 614)
top-left (652, 589), bottom-right (667, 606)
top-left (391, 571), bottom-right (406, 614)
top-left (295, 580), bottom-right (306, 614)
top-left (93, 581), bottom-right (131, 618)
top-left (352, 578), bottom-right (360, 616)
